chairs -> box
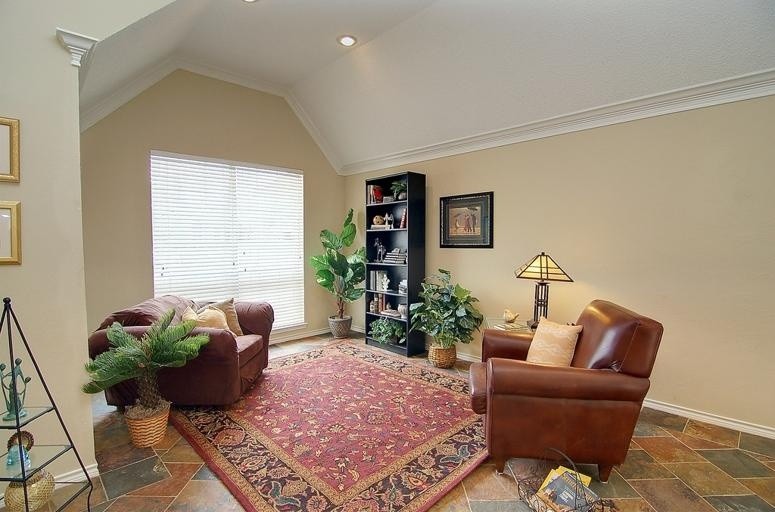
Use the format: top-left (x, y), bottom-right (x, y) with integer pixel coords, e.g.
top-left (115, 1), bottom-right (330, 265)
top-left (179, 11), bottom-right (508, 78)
top-left (468, 298), bottom-right (664, 485)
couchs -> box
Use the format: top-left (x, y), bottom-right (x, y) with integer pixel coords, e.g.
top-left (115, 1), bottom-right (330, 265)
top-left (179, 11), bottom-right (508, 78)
top-left (87, 293), bottom-right (275, 415)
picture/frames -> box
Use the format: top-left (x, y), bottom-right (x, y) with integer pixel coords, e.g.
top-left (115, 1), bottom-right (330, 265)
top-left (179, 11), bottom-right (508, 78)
top-left (0, 117), bottom-right (20, 182)
top-left (0, 200), bottom-right (22, 265)
top-left (439, 191), bottom-right (494, 249)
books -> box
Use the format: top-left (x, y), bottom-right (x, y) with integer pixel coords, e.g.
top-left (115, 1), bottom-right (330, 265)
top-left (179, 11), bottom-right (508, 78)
top-left (384, 247), bottom-right (407, 264)
top-left (367, 184), bottom-right (382, 203)
top-left (370, 270), bottom-right (388, 312)
top-left (493, 321), bottom-right (530, 331)
top-left (529, 466), bottom-right (592, 512)
top-left (400, 208), bottom-right (408, 228)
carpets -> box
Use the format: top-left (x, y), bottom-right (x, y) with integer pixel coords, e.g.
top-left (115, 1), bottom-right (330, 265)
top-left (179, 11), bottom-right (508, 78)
top-left (162, 339), bottom-right (489, 511)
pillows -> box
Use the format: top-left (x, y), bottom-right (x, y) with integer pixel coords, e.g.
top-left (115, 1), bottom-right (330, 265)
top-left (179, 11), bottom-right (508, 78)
top-left (182, 306), bottom-right (237, 338)
top-left (526, 316), bottom-right (583, 367)
top-left (204, 297), bottom-right (244, 336)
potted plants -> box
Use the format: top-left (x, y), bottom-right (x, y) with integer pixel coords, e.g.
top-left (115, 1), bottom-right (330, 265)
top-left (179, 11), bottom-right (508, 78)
top-left (83, 310), bottom-right (209, 446)
top-left (408, 267), bottom-right (482, 369)
top-left (308, 207), bottom-right (365, 338)
top-left (389, 179), bottom-right (407, 201)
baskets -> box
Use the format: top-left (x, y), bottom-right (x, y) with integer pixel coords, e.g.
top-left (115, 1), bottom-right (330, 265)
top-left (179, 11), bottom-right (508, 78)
top-left (518, 447), bottom-right (614, 512)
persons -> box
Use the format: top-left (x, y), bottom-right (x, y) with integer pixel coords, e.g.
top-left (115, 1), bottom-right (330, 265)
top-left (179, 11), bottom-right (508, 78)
top-left (455, 207), bottom-right (479, 232)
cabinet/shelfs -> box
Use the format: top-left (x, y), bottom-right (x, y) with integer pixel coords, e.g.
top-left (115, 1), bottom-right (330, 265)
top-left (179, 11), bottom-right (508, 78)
top-left (484, 316), bottom-right (537, 338)
top-left (0, 294), bottom-right (94, 512)
top-left (363, 169), bottom-right (427, 358)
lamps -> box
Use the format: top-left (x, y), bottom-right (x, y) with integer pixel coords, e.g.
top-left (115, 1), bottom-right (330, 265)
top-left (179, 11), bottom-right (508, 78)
top-left (514, 250), bottom-right (574, 323)
